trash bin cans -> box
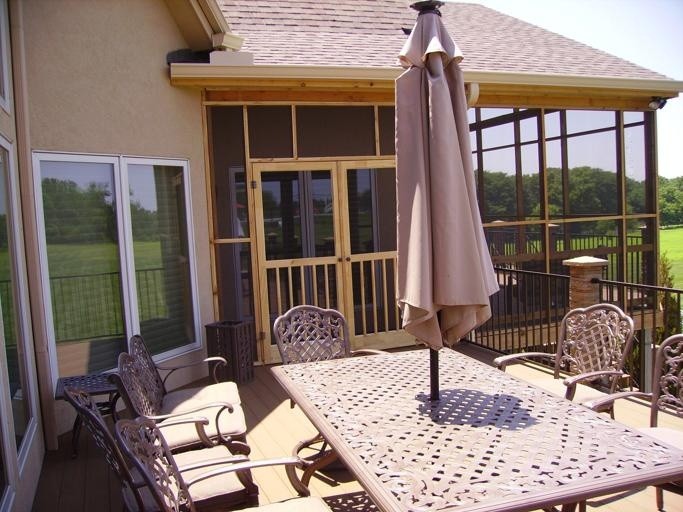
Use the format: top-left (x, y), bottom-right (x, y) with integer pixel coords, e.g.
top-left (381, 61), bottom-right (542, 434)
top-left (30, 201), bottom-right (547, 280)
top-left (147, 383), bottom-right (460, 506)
top-left (204, 320), bottom-right (254, 387)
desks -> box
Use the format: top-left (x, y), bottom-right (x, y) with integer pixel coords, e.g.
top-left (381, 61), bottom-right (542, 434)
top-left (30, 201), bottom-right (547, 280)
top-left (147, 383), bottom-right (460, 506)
top-left (270, 347), bottom-right (683, 512)
top-left (55, 372), bottom-right (122, 459)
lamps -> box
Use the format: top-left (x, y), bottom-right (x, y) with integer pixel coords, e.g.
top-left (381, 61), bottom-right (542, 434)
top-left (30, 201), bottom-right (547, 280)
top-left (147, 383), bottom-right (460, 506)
top-left (648, 96), bottom-right (668, 111)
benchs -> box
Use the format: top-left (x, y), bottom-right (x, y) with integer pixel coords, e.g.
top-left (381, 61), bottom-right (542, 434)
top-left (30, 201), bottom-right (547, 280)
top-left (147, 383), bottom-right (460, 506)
top-left (109, 352), bottom-right (250, 454)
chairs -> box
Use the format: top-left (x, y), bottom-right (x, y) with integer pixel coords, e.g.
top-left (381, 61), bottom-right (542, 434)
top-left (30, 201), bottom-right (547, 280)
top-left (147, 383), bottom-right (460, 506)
top-left (115, 416), bottom-right (334, 512)
top-left (274, 305), bottom-right (391, 484)
top-left (493, 303), bottom-right (635, 419)
top-left (580, 334), bottom-right (683, 512)
top-left (128, 334), bottom-right (242, 417)
top-left (60, 384), bottom-right (258, 511)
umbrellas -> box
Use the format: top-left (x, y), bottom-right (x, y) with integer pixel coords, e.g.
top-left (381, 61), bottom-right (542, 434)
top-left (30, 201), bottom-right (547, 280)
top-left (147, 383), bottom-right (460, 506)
top-left (392, 1), bottom-right (500, 401)
